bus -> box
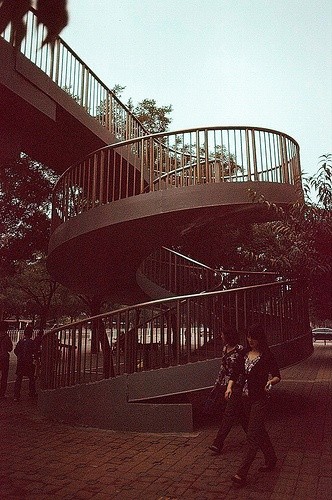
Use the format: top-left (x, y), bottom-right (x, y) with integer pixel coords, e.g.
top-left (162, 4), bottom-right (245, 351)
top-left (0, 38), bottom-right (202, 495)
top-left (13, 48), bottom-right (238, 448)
top-left (4, 320), bottom-right (34, 336)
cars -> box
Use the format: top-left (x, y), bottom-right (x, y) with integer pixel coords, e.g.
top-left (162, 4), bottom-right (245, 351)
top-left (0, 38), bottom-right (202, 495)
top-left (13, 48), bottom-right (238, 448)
top-left (312, 328), bottom-right (332, 342)
top-left (200, 324), bottom-right (213, 337)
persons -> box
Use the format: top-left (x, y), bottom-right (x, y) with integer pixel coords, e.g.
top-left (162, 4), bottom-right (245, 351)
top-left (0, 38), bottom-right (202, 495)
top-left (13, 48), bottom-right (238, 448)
top-left (224, 324), bottom-right (281, 484)
top-left (0, 322), bottom-right (125, 401)
top-left (207, 327), bottom-right (247, 453)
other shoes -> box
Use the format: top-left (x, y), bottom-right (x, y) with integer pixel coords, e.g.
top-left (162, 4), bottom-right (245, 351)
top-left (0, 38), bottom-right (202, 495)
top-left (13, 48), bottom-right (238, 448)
top-left (209, 444), bottom-right (222, 454)
top-left (259, 463), bottom-right (277, 472)
top-left (231, 472), bottom-right (248, 486)
